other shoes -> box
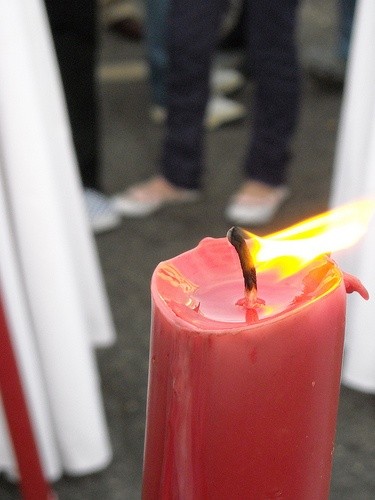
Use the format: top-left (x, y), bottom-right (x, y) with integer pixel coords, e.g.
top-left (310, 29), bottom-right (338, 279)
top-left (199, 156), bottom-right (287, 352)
top-left (154, 95), bottom-right (248, 128)
top-left (204, 67), bottom-right (246, 95)
top-left (309, 63), bottom-right (343, 87)
top-left (114, 179), bottom-right (200, 220)
top-left (83, 189), bottom-right (120, 232)
top-left (226, 182), bottom-right (293, 225)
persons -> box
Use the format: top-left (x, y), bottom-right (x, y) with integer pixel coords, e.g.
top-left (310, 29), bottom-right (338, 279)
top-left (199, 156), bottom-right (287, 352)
top-left (108, 0), bottom-right (308, 227)
top-left (43, 0), bottom-right (122, 235)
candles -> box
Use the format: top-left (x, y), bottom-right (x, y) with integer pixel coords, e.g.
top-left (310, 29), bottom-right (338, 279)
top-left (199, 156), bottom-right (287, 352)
top-left (136, 194), bottom-right (375, 500)
